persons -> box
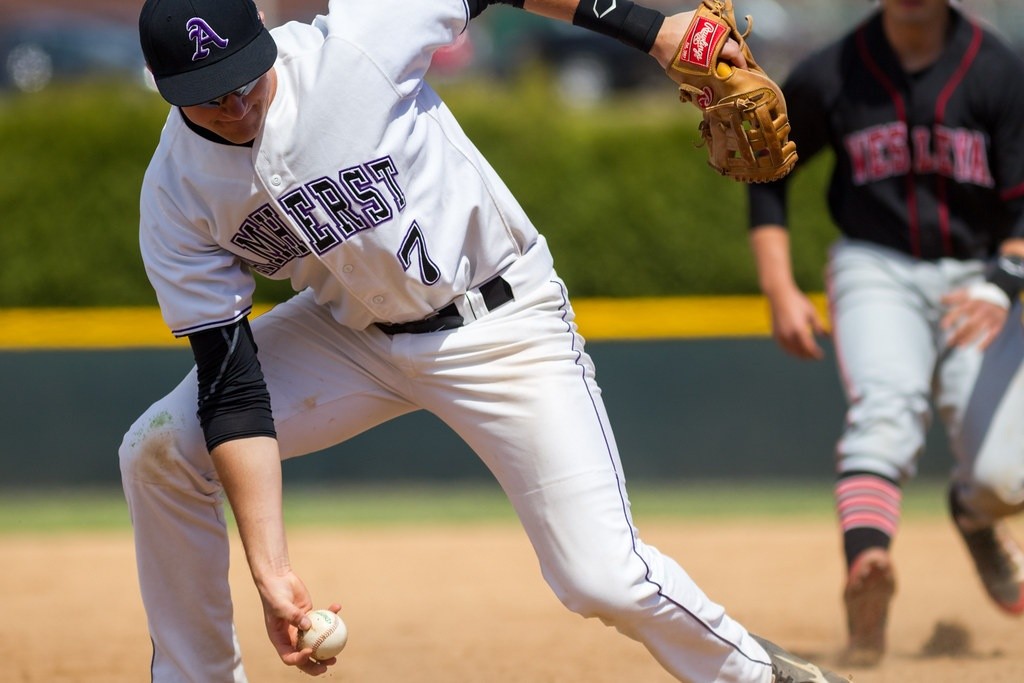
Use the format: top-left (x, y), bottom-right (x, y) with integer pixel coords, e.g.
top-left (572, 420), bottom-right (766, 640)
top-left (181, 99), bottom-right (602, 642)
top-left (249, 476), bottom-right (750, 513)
top-left (116, 0), bottom-right (851, 681)
top-left (743, 0), bottom-right (1024, 667)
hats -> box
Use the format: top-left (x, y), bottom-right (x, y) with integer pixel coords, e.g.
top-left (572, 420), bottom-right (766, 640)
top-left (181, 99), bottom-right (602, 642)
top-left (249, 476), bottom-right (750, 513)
top-left (138, 0), bottom-right (279, 108)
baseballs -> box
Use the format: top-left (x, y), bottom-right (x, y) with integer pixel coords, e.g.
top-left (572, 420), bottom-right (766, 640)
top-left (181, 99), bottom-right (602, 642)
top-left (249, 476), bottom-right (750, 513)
top-left (296, 608), bottom-right (350, 662)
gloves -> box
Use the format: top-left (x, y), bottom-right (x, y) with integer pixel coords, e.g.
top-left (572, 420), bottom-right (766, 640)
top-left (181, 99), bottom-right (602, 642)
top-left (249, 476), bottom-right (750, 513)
top-left (937, 252), bottom-right (1024, 360)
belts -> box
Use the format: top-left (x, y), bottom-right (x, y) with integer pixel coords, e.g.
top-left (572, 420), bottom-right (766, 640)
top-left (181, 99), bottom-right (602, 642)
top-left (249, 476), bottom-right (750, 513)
top-left (370, 275), bottom-right (523, 338)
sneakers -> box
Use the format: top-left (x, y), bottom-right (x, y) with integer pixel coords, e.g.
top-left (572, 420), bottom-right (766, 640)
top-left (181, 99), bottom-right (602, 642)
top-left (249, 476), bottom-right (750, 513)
top-left (937, 486), bottom-right (1024, 621)
top-left (840, 542), bottom-right (897, 670)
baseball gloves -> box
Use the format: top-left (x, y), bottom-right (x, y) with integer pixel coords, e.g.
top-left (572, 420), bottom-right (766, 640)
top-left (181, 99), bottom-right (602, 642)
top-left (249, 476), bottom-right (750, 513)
top-left (664, 0), bottom-right (799, 185)
